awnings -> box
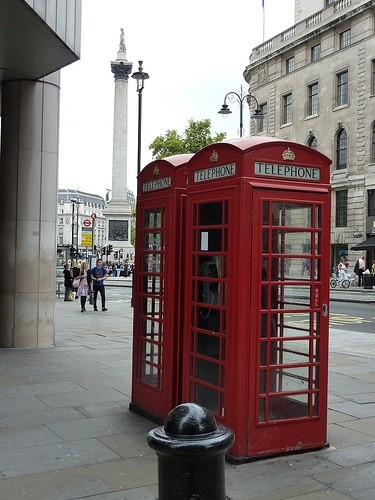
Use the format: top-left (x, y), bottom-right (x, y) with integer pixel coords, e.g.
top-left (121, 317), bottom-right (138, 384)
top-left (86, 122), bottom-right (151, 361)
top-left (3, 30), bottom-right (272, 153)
top-left (350, 237), bottom-right (375, 251)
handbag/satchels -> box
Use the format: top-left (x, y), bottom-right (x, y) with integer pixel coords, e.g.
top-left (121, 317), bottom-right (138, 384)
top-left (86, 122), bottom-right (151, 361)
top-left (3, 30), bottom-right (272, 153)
top-left (66, 291), bottom-right (75, 300)
top-left (76, 275), bottom-right (89, 296)
top-left (72, 277), bottom-right (80, 288)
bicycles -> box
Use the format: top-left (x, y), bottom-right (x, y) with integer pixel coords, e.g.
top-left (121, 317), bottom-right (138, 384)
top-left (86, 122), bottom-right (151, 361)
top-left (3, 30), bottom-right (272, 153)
top-left (331, 271), bottom-right (351, 289)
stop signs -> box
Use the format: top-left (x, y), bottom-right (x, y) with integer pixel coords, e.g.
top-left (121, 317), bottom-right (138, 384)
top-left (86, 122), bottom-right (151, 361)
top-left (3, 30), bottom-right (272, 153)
top-left (83, 220), bottom-right (91, 226)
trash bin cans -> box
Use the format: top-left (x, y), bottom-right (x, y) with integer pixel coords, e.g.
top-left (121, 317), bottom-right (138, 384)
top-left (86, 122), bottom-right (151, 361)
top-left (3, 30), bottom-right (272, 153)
top-left (363, 274), bottom-right (373, 289)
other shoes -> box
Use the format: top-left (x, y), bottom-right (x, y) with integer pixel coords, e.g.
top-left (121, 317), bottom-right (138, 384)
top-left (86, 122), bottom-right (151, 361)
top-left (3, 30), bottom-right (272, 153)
top-left (102, 309), bottom-right (108, 311)
top-left (94, 309), bottom-right (98, 311)
top-left (81, 309), bottom-right (86, 312)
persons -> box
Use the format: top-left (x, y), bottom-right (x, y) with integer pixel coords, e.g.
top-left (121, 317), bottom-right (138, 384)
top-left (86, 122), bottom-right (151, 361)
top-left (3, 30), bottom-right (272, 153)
top-left (104, 258), bottom-right (133, 278)
top-left (338, 255), bottom-right (351, 287)
top-left (75, 261), bottom-right (92, 312)
top-left (301, 258), bottom-right (310, 277)
top-left (63, 264), bottom-right (74, 301)
top-left (354, 256), bottom-right (364, 287)
top-left (90, 259), bottom-right (108, 311)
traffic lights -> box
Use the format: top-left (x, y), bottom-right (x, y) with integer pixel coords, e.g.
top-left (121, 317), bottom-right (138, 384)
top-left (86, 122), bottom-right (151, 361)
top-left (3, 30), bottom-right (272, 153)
top-left (93, 245), bottom-right (97, 251)
top-left (120, 253), bottom-right (123, 258)
top-left (114, 251), bottom-right (118, 258)
top-left (109, 244), bottom-right (113, 250)
top-left (106, 246), bottom-right (111, 255)
top-left (102, 247), bottom-right (106, 255)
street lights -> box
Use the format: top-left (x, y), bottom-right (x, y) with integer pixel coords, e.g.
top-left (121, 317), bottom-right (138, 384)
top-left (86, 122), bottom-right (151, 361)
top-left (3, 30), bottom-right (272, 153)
top-left (130, 59), bottom-right (150, 176)
top-left (70, 199), bottom-right (77, 266)
top-left (217, 83), bottom-right (264, 138)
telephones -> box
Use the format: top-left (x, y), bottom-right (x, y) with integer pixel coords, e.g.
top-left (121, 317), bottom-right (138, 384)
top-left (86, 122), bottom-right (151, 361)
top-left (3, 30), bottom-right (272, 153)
top-left (202, 257), bottom-right (220, 305)
